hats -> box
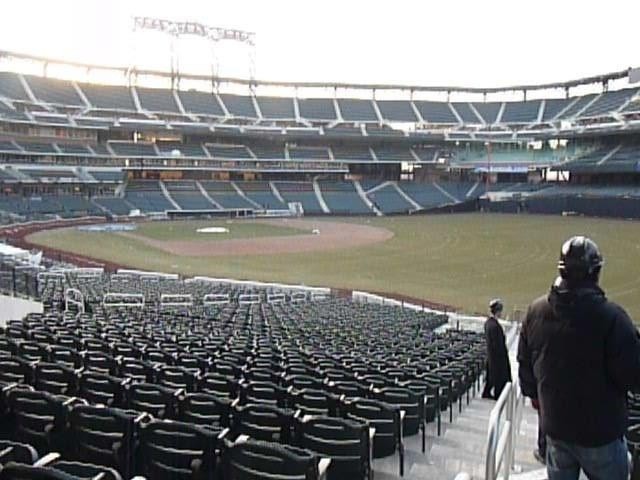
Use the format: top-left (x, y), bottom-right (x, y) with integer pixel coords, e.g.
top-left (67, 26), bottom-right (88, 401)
top-left (490, 300), bottom-right (502, 312)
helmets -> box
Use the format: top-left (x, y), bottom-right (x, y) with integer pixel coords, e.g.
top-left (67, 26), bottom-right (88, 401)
top-left (558, 236), bottom-right (602, 279)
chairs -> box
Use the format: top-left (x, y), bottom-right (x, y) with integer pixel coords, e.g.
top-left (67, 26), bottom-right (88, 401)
top-left (0, 256), bottom-right (505, 480)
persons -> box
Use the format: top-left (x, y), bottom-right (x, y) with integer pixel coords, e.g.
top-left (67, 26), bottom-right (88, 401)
top-left (534, 411), bottom-right (554, 464)
top-left (482, 298), bottom-right (512, 401)
top-left (626, 329), bottom-right (640, 480)
top-left (518, 235), bottom-right (640, 480)
